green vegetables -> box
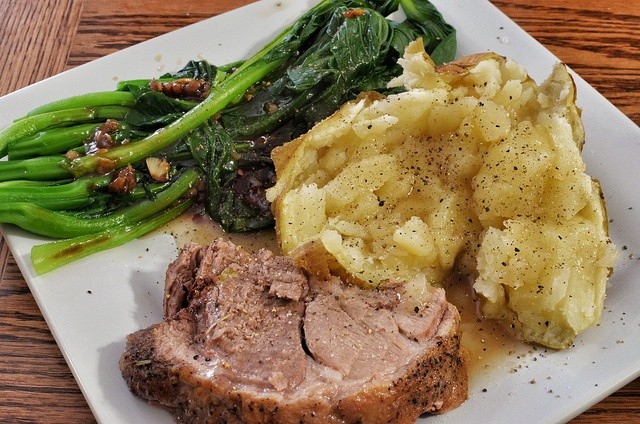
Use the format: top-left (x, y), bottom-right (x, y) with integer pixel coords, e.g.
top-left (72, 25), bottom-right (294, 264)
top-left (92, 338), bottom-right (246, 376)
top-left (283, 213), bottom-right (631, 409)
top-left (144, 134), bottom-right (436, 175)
top-left (0, 0), bottom-right (456, 277)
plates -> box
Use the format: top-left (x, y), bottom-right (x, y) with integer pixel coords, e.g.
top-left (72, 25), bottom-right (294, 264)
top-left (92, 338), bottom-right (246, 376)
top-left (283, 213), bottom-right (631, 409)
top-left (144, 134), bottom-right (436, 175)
top-left (0, 0), bottom-right (640, 424)
